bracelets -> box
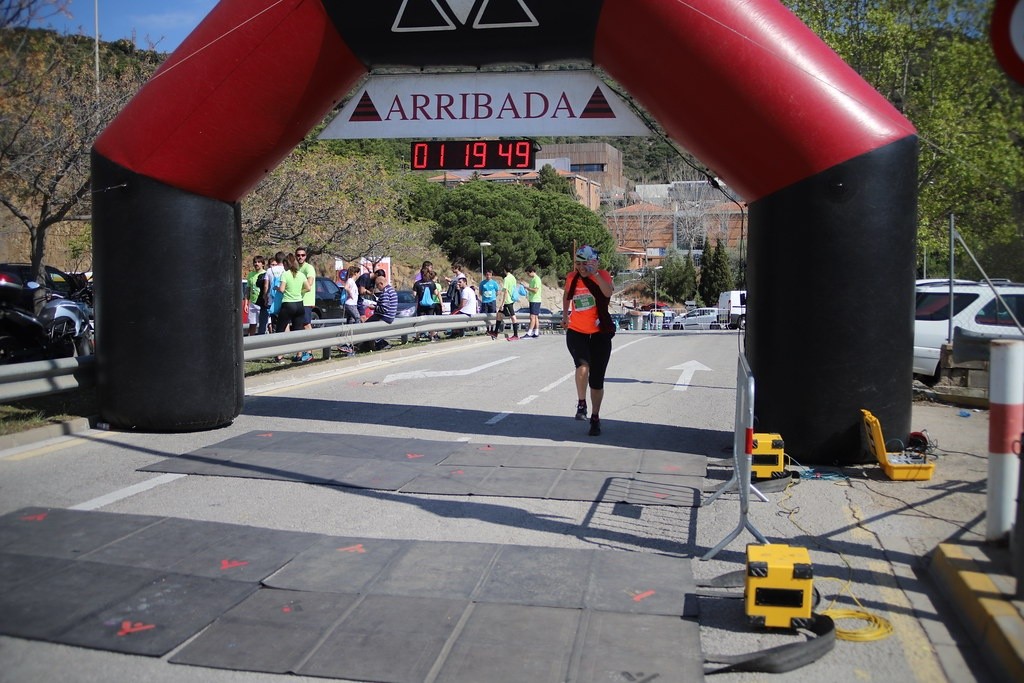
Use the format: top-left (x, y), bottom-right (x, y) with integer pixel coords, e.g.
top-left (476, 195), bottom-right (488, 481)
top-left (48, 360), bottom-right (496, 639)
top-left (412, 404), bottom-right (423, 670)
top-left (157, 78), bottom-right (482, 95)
top-left (594, 271), bottom-right (599, 277)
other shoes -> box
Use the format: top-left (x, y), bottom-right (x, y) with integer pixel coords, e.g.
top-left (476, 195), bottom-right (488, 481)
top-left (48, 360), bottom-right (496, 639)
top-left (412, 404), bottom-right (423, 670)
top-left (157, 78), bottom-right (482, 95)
top-left (445, 333), bottom-right (465, 340)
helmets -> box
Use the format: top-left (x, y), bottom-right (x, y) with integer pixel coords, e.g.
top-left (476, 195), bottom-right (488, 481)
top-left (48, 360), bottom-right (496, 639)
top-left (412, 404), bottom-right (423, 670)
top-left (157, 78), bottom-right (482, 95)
top-left (574, 246), bottom-right (601, 262)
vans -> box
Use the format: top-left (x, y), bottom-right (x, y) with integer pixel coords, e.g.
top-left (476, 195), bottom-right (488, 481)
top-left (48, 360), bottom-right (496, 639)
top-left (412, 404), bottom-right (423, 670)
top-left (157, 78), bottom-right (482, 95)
top-left (718, 291), bottom-right (747, 330)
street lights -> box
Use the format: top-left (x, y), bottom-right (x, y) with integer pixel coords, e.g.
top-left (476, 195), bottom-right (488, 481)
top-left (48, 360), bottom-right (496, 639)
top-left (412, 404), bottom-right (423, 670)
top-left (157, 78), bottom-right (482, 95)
top-left (654, 266), bottom-right (664, 311)
top-left (479, 242), bottom-right (491, 280)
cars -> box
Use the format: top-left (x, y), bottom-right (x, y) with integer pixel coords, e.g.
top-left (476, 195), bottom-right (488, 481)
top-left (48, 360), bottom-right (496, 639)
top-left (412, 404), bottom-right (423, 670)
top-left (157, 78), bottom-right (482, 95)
top-left (396, 289), bottom-right (572, 318)
top-left (307, 277), bottom-right (362, 328)
top-left (662, 310), bottom-right (677, 329)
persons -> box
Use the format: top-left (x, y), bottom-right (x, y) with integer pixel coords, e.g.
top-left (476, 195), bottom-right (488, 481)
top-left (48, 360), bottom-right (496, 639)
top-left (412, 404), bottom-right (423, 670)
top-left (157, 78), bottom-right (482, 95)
top-left (338, 266), bottom-right (398, 353)
top-left (243, 247), bottom-right (316, 363)
top-left (412, 261), bottom-right (541, 341)
top-left (560, 245), bottom-right (613, 436)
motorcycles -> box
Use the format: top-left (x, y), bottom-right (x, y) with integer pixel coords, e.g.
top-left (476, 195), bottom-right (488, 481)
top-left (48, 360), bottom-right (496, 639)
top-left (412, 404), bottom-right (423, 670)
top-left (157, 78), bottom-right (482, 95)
top-left (0, 270), bottom-right (95, 364)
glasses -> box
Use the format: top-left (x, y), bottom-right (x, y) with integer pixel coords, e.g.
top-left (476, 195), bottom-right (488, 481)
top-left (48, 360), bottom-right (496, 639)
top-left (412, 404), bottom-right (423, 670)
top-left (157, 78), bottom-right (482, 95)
top-left (297, 254), bottom-right (306, 257)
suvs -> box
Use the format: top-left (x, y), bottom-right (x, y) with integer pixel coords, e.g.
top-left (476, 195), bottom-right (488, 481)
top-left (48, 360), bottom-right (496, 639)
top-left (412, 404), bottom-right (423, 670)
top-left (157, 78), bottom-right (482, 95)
top-left (914, 279), bottom-right (1024, 388)
top-left (0, 262), bottom-right (81, 316)
top-left (673, 308), bottom-right (730, 329)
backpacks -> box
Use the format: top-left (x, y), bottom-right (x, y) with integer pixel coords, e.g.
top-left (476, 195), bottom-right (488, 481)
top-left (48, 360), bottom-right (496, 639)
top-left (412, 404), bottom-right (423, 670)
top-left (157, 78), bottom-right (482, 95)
top-left (340, 288), bottom-right (350, 306)
top-left (508, 276), bottom-right (527, 304)
top-left (266, 268), bottom-right (285, 317)
top-left (419, 280), bottom-right (435, 306)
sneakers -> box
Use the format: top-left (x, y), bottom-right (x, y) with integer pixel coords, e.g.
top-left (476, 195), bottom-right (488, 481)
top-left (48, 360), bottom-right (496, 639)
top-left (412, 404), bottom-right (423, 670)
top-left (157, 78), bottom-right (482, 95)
top-left (414, 337), bottom-right (421, 343)
top-left (302, 351), bottom-right (314, 363)
top-left (575, 406), bottom-right (588, 421)
top-left (486, 330), bottom-right (498, 339)
top-left (291, 356), bottom-right (303, 364)
top-left (337, 343), bottom-right (354, 354)
top-left (267, 356), bottom-right (285, 364)
top-left (507, 335), bottom-right (518, 341)
top-left (520, 334), bottom-right (539, 340)
top-left (429, 332), bottom-right (439, 343)
top-left (588, 421), bottom-right (602, 437)
